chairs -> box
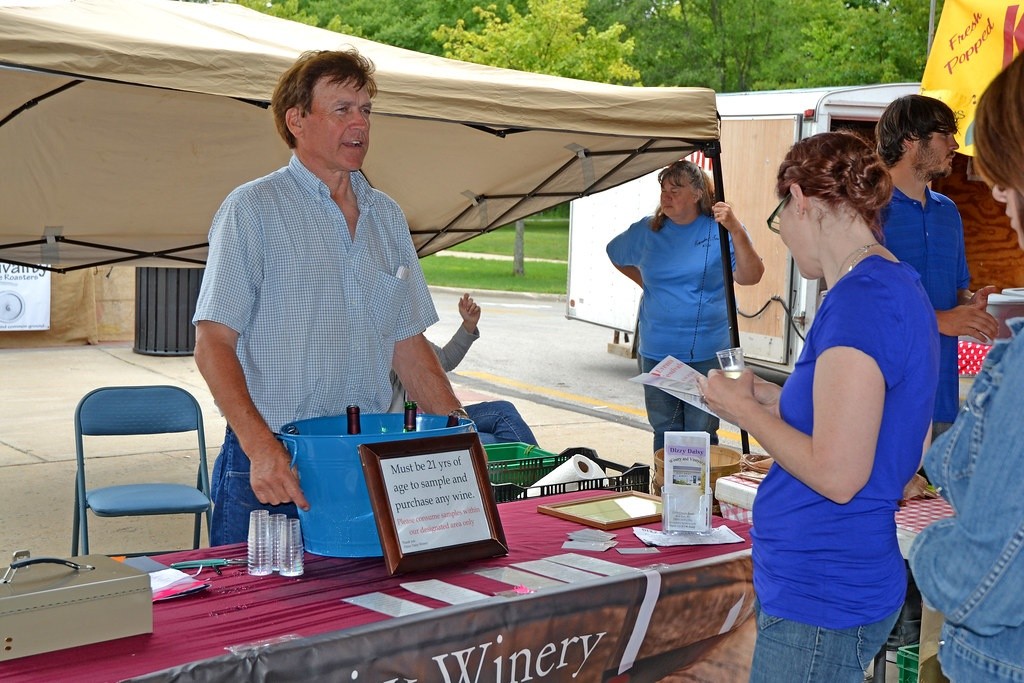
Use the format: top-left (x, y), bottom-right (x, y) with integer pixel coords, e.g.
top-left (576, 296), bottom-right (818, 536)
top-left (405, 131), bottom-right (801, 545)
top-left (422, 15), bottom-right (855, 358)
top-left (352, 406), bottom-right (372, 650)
top-left (71, 385), bottom-right (214, 555)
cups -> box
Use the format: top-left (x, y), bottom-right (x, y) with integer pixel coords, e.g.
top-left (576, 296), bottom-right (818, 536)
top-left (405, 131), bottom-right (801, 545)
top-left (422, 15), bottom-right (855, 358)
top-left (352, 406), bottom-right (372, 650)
top-left (716, 348), bottom-right (745, 379)
top-left (247, 510), bottom-right (305, 576)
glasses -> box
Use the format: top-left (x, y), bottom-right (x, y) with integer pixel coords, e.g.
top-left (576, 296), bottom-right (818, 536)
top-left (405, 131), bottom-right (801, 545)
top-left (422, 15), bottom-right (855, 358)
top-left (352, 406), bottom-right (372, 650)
top-left (766, 188), bottom-right (792, 234)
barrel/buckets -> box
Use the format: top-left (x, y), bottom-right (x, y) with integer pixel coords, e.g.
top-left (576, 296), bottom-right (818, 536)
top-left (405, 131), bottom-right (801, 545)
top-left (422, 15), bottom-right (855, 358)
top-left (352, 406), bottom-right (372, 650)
top-left (275, 413), bottom-right (478, 557)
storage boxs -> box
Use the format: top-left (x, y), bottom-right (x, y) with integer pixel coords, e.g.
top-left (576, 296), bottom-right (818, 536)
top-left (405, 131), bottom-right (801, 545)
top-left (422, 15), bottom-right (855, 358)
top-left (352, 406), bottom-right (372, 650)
top-left (896, 643), bottom-right (920, 683)
top-left (483, 441), bottom-right (651, 504)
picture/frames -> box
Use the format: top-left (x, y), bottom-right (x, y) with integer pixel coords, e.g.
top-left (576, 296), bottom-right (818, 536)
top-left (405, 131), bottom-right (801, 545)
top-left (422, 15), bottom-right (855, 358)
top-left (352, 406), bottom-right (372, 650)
top-left (537, 490), bottom-right (662, 531)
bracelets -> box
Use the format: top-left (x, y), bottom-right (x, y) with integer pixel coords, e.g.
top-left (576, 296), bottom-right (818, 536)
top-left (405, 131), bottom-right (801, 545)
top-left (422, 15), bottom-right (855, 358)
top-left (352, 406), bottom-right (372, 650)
top-left (447, 406), bottom-right (468, 417)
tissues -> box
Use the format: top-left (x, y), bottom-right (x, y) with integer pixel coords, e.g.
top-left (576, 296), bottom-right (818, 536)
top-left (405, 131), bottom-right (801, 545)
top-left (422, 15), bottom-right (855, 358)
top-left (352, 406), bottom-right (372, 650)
top-left (714, 470), bottom-right (767, 511)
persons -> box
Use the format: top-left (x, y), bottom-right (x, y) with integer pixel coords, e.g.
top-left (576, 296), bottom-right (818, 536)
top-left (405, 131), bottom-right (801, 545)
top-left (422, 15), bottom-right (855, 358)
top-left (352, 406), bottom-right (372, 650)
top-left (606, 161), bottom-right (764, 472)
top-left (908, 50), bottom-right (1023, 683)
top-left (874, 95), bottom-right (999, 665)
top-left (194, 50), bottom-right (487, 547)
top-left (387, 294), bottom-right (542, 450)
top-left (701, 133), bottom-right (941, 683)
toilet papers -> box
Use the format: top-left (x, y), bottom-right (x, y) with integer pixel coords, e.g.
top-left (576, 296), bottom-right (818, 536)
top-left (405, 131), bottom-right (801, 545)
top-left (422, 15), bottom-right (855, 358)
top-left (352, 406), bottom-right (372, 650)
top-left (511, 453), bottom-right (609, 500)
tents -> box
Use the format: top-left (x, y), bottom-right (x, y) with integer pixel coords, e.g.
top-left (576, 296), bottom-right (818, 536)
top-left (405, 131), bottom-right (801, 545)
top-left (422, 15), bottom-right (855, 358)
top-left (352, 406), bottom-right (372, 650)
top-left (0, 0), bottom-right (750, 456)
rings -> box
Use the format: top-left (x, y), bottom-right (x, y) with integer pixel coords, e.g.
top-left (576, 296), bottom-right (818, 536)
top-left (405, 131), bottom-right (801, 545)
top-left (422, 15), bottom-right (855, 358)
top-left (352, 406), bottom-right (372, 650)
top-left (701, 393), bottom-right (706, 401)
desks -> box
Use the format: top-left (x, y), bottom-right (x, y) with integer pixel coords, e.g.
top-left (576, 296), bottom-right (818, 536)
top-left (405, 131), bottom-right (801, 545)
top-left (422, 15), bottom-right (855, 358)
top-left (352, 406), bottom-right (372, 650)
top-left (0, 491), bottom-right (758, 683)
top-left (715, 470), bottom-right (953, 683)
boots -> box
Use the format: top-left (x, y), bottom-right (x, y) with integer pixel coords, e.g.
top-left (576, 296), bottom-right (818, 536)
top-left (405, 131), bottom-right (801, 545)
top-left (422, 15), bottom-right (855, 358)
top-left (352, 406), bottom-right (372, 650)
top-left (884, 606), bottom-right (921, 651)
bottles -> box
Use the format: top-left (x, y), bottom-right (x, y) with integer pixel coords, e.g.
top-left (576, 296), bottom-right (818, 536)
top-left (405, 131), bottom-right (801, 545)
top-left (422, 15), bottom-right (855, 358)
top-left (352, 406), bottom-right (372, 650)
top-left (283, 425), bottom-right (300, 435)
top-left (403, 401), bottom-right (417, 432)
top-left (446, 411), bottom-right (460, 428)
top-left (347, 405), bottom-right (361, 435)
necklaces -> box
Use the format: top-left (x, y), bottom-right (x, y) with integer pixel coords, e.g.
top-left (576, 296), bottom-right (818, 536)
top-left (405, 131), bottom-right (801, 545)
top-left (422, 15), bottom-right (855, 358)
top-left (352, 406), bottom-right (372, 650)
top-left (820, 243), bottom-right (880, 299)
